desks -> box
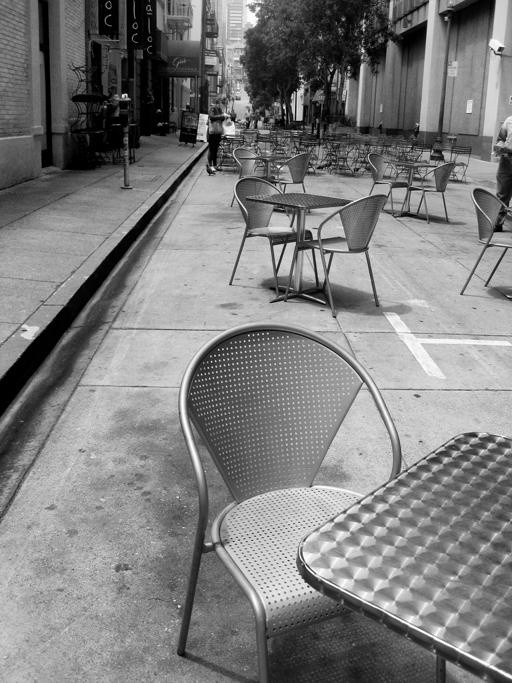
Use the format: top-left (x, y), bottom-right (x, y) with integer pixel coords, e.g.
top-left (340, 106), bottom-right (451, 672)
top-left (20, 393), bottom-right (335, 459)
top-left (240, 154), bottom-right (292, 180)
top-left (244, 193), bottom-right (353, 305)
top-left (296, 428), bottom-right (510, 682)
top-left (384, 161), bottom-right (437, 221)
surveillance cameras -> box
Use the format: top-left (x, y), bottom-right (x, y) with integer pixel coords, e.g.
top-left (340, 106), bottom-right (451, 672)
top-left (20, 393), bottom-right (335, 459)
top-left (488, 38), bottom-right (504, 55)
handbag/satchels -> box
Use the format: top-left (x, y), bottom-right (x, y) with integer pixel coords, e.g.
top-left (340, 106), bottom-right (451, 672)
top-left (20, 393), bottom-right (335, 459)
top-left (209, 119), bottom-right (222, 134)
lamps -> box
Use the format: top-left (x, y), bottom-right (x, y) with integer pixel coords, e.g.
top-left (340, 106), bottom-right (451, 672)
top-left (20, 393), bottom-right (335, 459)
top-left (402, 16), bottom-right (412, 28)
top-left (489, 38), bottom-right (506, 56)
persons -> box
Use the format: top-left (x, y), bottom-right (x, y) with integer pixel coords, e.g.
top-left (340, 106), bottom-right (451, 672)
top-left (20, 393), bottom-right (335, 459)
top-left (491, 113), bottom-right (512, 232)
top-left (224, 107), bottom-right (286, 131)
top-left (204, 93), bottom-right (233, 173)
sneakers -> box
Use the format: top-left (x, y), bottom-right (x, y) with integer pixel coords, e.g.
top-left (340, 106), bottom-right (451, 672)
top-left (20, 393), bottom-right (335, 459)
top-left (211, 165), bottom-right (223, 172)
top-left (495, 224), bottom-right (503, 231)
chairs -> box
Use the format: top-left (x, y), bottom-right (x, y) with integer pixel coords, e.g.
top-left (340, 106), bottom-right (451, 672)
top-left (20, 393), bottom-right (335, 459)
top-left (227, 174), bottom-right (292, 298)
top-left (230, 146), bottom-right (265, 209)
top-left (458, 187), bottom-right (511, 297)
top-left (301, 191), bottom-right (391, 317)
top-left (176, 321), bottom-right (404, 682)
top-left (278, 151), bottom-right (312, 194)
top-left (366, 152), bottom-right (399, 211)
top-left (406, 158), bottom-right (458, 224)
